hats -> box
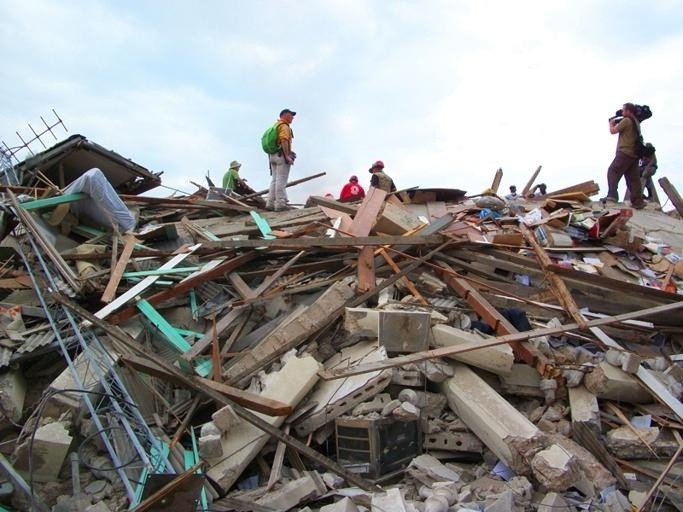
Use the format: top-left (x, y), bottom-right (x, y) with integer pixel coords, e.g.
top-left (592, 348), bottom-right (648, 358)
top-left (280, 109), bottom-right (296, 117)
top-left (349, 176), bottom-right (358, 182)
top-left (229, 161), bottom-right (241, 168)
top-left (369, 160), bottom-right (384, 172)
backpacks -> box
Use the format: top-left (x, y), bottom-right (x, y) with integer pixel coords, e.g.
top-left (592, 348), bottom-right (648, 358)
top-left (261, 122), bottom-right (282, 154)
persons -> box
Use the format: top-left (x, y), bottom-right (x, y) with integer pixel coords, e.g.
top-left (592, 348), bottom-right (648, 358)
top-left (369, 161), bottom-right (397, 193)
top-left (222, 161), bottom-right (257, 194)
top-left (505, 186), bottom-right (522, 199)
top-left (340, 176), bottom-right (365, 200)
top-left (600, 103), bottom-right (645, 209)
top-left (639, 143), bottom-right (658, 199)
top-left (264, 110), bottom-right (296, 212)
top-left (531, 184), bottom-right (546, 196)
